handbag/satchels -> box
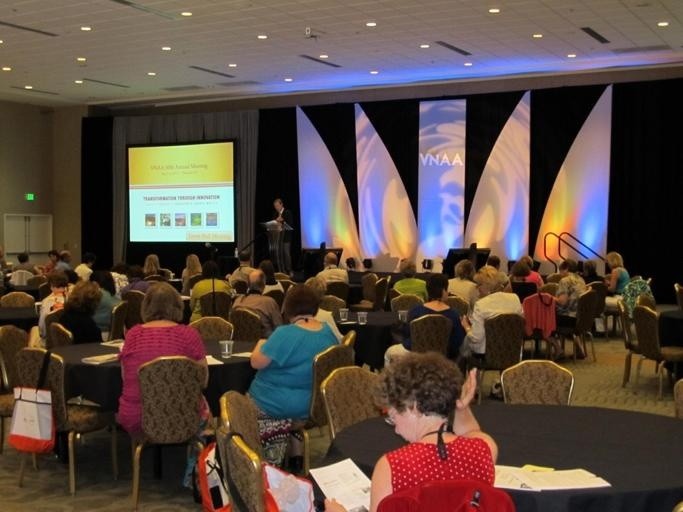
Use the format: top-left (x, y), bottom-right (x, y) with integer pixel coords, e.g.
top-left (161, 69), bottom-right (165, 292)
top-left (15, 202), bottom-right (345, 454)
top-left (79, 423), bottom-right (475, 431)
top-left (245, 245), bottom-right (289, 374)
top-left (7, 351), bottom-right (56, 453)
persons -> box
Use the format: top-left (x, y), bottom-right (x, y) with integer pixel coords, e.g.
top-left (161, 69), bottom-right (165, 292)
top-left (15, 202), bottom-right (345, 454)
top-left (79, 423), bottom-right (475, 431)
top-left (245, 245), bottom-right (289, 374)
top-left (114, 281), bottom-right (214, 491)
top-left (243, 282), bottom-right (338, 474)
top-left (273, 199), bottom-right (294, 273)
top-left (1, 248), bottom-right (349, 343)
top-left (323, 351), bottom-right (497, 512)
top-left (393, 252), bottom-right (629, 356)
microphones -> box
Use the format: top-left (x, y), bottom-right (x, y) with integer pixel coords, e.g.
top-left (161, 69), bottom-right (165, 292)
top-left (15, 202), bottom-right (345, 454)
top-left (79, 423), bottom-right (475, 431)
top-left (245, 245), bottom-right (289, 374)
top-left (204, 240), bottom-right (220, 254)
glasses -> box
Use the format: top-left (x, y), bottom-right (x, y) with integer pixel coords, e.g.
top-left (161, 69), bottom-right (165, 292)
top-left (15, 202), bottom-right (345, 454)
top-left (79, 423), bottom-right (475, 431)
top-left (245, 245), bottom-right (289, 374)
top-left (384, 410), bottom-right (401, 426)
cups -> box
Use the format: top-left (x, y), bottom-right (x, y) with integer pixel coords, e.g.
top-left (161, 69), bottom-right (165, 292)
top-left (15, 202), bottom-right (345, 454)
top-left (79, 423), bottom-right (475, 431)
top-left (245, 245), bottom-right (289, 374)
top-left (339, 308), bottom-right (350, 321)
top-left (357, 310), bottom-right (368, 325)
top-left (219, 340), bottom-right (234, 358)
top-left (397, 309), bottom-right (408, 320)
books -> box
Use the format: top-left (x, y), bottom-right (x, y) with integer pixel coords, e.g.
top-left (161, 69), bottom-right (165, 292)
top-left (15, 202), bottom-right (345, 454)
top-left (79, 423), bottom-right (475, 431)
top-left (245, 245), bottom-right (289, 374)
top-left (309, 457), bottom-right (371, 511)
